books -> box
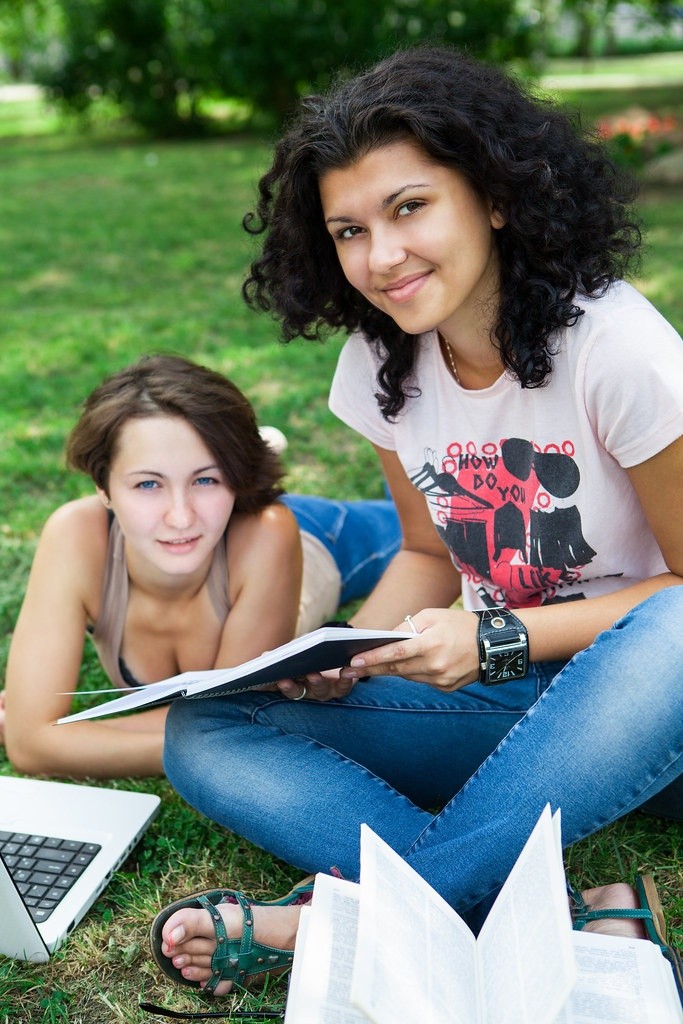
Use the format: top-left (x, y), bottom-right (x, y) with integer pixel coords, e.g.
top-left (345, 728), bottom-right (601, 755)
top-left (53, 624), bottom-right (420, 730)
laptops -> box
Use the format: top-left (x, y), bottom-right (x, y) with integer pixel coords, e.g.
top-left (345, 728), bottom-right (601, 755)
top-left (0, 775), bottom-right (161, 963)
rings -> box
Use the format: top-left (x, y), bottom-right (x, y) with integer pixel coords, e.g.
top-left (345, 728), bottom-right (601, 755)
top-left (404, 614), bottom-right (417, 634)
top-left (291, 687), bottom-right (306, 701)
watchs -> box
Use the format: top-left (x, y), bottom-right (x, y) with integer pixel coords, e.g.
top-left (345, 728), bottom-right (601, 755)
top-left (472, 606), bottom-right (530, 685)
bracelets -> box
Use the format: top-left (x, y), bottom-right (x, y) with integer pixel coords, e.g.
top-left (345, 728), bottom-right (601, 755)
top-left (320, 620), bottom-right (371, 683)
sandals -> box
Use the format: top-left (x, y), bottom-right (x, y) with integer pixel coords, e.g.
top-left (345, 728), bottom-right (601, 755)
top-left (149, 873), bottom-right (316, 998)
top-left (568, 873), bottom-right (683, 1008)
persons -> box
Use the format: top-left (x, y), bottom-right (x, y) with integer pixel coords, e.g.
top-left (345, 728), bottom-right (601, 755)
top-left (150, 42), bottom-right (682, 1024)
top-left (0, 353), bottom-right (408, 777)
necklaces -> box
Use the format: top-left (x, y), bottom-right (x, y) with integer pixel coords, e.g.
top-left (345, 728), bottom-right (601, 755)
top-left (445, 339), bottom-right (461, 387)
